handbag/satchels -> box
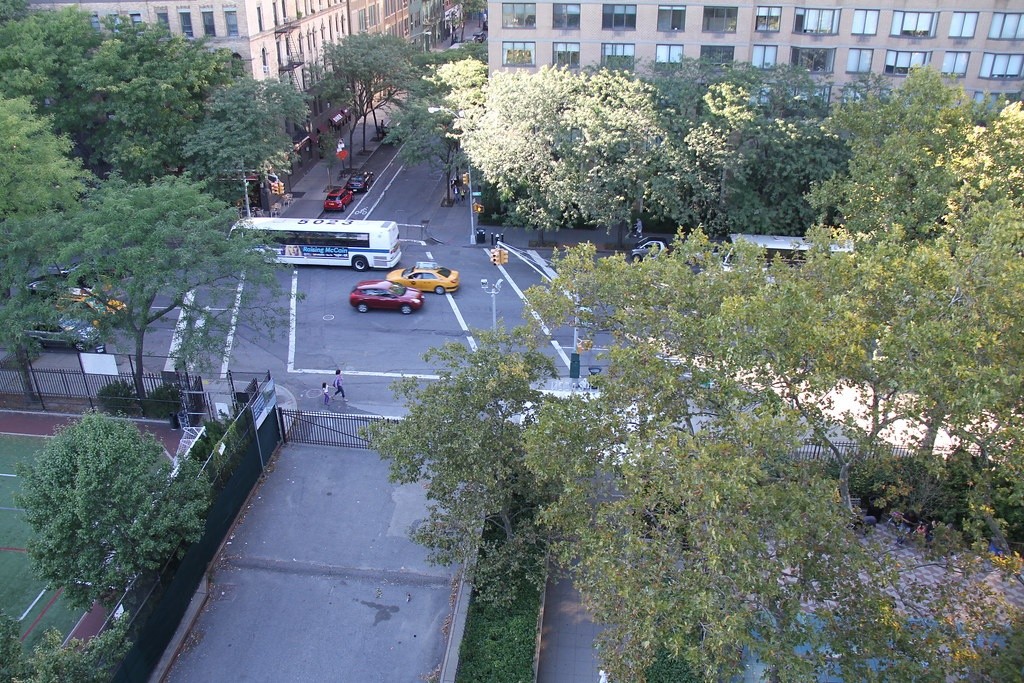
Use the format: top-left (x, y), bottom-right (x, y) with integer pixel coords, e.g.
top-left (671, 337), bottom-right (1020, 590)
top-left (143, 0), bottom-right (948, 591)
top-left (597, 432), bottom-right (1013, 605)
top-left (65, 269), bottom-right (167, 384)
top-left (332, 380), bottom-right (340, 387)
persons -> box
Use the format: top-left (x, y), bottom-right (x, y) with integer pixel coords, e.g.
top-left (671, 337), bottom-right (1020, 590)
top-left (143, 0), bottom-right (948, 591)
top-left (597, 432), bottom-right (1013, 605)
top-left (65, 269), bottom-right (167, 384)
top-left (633, 217), bottom-right (644, 239)
top-left (380, 118), bottom-right (387, 138)
top-left (453, 183), bottom-right (467, 204)
top-left (892, 509), bottom-right (954, 549)
top-left (322, 369), bottom-right (349, 406)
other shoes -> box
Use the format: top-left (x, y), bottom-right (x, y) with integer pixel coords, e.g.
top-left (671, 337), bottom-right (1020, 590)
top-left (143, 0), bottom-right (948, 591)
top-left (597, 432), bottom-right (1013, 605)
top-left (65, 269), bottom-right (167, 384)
top-left (324, 403), bottom-right (330, 406)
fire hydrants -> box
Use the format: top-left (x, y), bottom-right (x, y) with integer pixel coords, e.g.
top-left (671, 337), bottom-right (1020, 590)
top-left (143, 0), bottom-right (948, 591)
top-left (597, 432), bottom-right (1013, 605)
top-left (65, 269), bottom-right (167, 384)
top-left (495, 234), bottom-right (500, 244)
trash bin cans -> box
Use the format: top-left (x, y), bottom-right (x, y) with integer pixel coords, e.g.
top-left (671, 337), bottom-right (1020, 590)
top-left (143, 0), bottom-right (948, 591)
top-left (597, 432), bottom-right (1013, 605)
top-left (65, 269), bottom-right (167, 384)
top-left (569, 352), bottom-right (580, 378)
top-left (476, 227), bottom-right (486, 243)
top-left (588, 365), bottom-right (601, 390)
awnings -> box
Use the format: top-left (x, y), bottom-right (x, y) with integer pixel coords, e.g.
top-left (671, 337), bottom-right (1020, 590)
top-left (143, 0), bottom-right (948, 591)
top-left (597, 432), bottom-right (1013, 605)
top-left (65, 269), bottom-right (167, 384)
top-left (198, 169), bottom-right (259, 181)
top-left (308, 104), bottom-right (354, 144)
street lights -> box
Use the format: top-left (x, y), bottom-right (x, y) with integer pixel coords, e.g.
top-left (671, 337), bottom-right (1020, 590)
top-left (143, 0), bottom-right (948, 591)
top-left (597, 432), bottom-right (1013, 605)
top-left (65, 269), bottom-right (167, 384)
top-left (337, 139), bottom-right (347, 178)
top-left (430, 106), bottom-right (477, 246)
top-left (481, 276), bottom-right (503, 348)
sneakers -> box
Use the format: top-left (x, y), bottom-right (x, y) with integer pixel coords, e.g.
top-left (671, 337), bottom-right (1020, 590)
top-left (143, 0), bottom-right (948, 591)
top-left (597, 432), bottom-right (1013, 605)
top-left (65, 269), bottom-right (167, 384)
top-left (342, 399), bottom-right (348, 401)
top-left (329, 396), bottom-right (335, 400)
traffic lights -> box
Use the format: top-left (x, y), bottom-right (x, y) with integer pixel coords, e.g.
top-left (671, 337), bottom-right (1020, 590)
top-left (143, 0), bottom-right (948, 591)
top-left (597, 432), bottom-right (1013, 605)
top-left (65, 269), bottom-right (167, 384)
top-left (473, 205), bottom-right (485, 213)
top-left (461, 174), bottom-right (469, 185)
top-left (278, 183), bottom-right (285, 195)
top-left (270, 183), bottom-right (280, 195)
top-left (489, 248), bottom-right (500, 267)
top-left (501, 249), bottom-right (510, 265)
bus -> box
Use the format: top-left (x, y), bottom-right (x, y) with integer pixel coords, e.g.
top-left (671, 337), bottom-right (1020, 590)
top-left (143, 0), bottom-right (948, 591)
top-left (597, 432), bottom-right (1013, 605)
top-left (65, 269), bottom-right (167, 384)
top-left (226, 216), bottom-right (402, 271)
top-left (212, 167), bottom-right (285, 207)
top-left (716, 233), bottom-right (852, 274)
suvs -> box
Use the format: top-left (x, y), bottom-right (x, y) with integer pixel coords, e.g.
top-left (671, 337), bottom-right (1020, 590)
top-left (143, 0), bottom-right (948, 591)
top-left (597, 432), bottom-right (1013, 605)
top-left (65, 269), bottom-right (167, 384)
top-left (349, 280), bottom-right (425, 314)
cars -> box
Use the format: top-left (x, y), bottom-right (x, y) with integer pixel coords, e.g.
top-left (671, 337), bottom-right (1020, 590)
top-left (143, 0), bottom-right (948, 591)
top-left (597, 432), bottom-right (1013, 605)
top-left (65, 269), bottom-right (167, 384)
top-left (385, 266), bottom-right (461, 295)
top-left (348, 172), bottom-right (374, 191)
top-left (22, 316), bottom-right (102, 349)
top-left (22, 260), bottom-right (127, 296)
top-left (634, 236), bottom-right (668, 247)
top-left (324, 189), bottom-right (355, 212)
top-left (687, 248), bottom-right (719, 265)
top-left (482, 21), bottom-right (488, 31)
top-left (472, 32), bottom-right (487, 42)
top-left (53, 286), bottom-right (129, 325)
top-left (631, 241), bottom-right (669, 263)
